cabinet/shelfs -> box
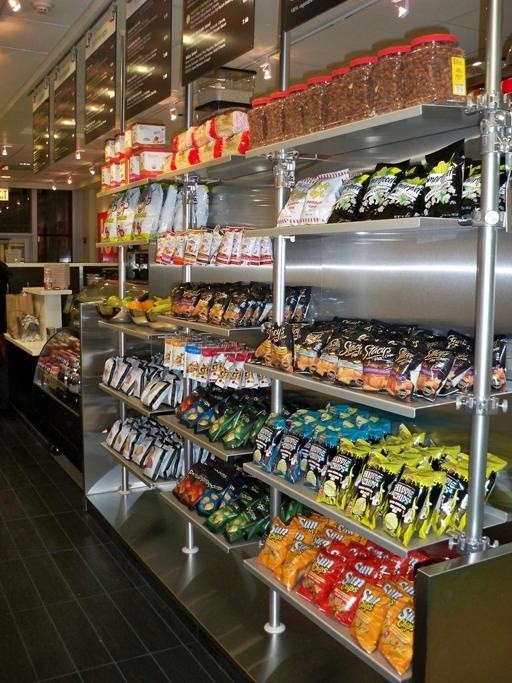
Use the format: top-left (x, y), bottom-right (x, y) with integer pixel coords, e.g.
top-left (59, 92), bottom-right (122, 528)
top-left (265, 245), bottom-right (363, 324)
top-left (4, 98), bottom-right (510, 683)
